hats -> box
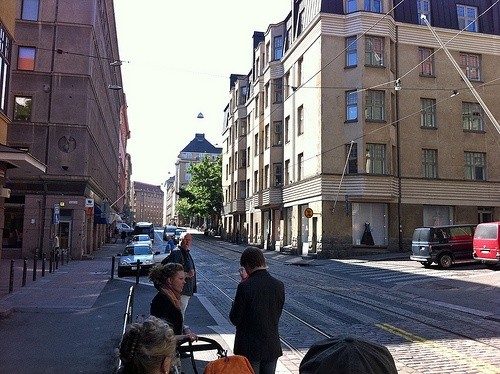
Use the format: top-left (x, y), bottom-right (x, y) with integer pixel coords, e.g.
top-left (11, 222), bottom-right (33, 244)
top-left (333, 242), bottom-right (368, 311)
top-left (299, 336), bottom-right (399, 374)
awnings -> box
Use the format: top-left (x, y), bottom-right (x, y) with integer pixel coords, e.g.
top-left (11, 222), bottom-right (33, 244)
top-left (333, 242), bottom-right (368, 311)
top-left (95, 206), bottom-right (109, 224)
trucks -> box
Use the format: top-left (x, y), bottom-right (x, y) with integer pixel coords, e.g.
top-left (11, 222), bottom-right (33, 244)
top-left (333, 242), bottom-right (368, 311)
top-left (134, 222), bottom-right (155, 243)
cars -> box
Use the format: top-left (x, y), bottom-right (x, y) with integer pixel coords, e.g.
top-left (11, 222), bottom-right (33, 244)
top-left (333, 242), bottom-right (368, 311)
top-left (130, 234), bottom-right (154, 250)
top-left (117, 245), bottom-right (160, 277)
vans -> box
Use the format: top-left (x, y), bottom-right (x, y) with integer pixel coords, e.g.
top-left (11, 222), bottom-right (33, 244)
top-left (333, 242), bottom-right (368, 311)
top-left (174, 228), bottom-right (188, 244)
top-left (410, 224), bottom-right (479, 269)
top-left (163, 226), bottom-right (177, 241)
top-left (472, 222), bottom-right (500, 270)
top-left (116, 223), bottom-right (134, 233)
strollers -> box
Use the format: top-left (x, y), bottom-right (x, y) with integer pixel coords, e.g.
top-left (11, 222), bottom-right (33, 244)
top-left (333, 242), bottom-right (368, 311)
top-left (177, 335), bottom-right (255, 374)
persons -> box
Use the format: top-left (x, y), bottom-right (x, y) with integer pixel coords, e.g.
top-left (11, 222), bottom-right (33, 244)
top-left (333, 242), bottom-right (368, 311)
top-left (229, 246), bottom-right (285, 374)
top-left (120, 315), bottom-right (179, 374)
top-left (168, 235), bottom-right (174, 253)
top-left (148, 262), bottom-right (198, 374)
top-left (167, 232), bottom-right (197, 329)
top-left (52, 232), bottom-right (60, 256)
top-left (121, 229), bottom-right (127, 243)
top-left (360, 220), bottom-right (375, 245)
top-left (114, 226), bottom-right (119, 243)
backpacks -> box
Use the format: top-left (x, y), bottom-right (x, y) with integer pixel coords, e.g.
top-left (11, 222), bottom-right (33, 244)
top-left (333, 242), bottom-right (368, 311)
top-left (161, 246), bottom-right (188, 267)
